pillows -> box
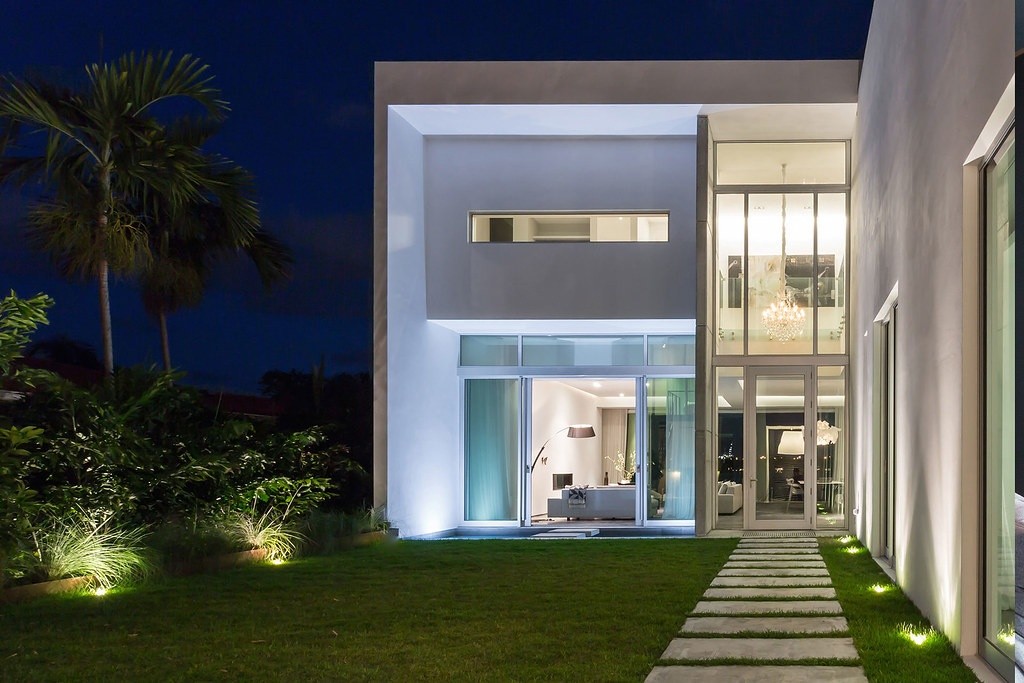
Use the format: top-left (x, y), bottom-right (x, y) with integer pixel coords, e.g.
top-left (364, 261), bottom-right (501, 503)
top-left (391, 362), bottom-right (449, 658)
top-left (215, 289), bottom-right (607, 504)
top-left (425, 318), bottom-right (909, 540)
top-left (718, 480), bottom-right (730, 493)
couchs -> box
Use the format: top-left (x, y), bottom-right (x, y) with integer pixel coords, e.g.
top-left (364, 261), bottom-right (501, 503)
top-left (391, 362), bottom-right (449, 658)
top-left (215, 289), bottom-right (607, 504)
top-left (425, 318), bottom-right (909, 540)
top-left (718, 480), bottom-right (743, 515)
top-left (547, 485), bottom-right (659, 521)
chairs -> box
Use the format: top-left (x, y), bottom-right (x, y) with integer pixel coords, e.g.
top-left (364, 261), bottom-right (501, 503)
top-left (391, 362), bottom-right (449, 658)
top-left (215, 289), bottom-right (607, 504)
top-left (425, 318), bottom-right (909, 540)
top-left (784, 478), bottom-right (804, 513)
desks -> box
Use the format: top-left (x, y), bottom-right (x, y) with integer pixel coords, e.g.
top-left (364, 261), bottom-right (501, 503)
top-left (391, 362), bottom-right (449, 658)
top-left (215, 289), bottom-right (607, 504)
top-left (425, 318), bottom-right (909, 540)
top-left (817, 477), bottom-right (844, 518)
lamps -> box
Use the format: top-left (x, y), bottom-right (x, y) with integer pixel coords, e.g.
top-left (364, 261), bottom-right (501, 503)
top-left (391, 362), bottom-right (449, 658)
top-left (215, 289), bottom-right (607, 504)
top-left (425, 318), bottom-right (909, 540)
top-left (777, 431), bottom-right (804, 455)
top-left (761, 165), bottom-right (805, 344)
top-left (816, 420), bottom-right (842, 446)
top-left (531, 424), bottom-right (596, 473)
